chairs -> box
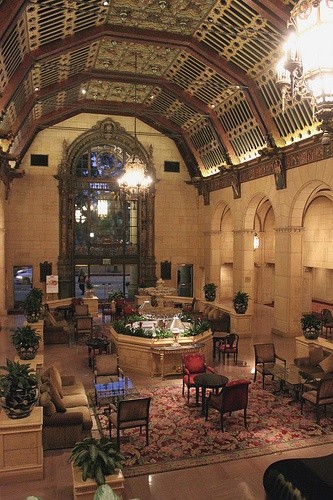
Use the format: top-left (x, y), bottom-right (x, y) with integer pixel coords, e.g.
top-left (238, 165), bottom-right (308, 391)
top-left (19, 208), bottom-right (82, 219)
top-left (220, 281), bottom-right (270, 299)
top-left (301, 379), bottom-right (333, 418)
top-left (92, 353), bottom-right (152, 450)
top-left (321, 308), bottom-right (333, 338)
top-left (253, 343), bottom-right (286, 389)
top-left (218, 333), bottom-right (239, 365)
top-left (181, 352), bottom-right (251, 433)
top-left (72, 304), bottom-right (94, 345)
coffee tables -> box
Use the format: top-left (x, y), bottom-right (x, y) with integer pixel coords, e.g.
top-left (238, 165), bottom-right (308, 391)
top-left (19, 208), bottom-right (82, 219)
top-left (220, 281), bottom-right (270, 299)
top-left (265, 363), bottom-right (321, 405)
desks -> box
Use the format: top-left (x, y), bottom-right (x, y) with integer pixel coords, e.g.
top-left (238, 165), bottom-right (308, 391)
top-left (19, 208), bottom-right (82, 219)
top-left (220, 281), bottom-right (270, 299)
top-left (193, 373), bottom-right (229, 414)
top-left (212, 331), bottom-right (230, 360)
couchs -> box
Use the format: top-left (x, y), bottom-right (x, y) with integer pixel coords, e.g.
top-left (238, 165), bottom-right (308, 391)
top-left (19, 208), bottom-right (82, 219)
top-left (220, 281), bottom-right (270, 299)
top-left (43, 311), bottom-right (70, 344)
top-left (182, 300), bottom-right (230, 334)
top-left (41, 366), bottom-right (92, 451)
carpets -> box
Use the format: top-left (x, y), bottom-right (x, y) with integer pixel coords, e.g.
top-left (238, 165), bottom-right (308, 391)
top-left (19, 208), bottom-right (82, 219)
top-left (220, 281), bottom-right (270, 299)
top-left (84, 378), bottom-right (333, 479)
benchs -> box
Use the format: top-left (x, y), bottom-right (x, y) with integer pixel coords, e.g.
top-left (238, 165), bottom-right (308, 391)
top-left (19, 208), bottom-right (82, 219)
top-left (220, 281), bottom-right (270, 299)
top-left (294, 350), bottom-right (333, 380)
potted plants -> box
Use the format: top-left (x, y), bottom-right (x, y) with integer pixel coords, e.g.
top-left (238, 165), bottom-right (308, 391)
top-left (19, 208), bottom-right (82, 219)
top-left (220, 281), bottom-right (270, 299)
top-left (108, 289), bottom-right (127, 307)
top-left (0, 290), bottom-right (42, 419)
top-left (202, 283), bottom-right (218, 302)
top-left (302, 315), bottom-right (321, 340)
top-left (231, 290), bottom-right (252, 314)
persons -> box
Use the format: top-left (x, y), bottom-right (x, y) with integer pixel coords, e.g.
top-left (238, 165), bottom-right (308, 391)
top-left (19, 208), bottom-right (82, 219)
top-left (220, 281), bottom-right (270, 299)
top-left (78, 271), bottom-right (86, 295)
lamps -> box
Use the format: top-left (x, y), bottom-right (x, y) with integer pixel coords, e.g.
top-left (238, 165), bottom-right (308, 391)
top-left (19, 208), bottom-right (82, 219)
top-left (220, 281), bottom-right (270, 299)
top-left (117, 52), bottom-right (157, 209)
top-left (273, 0), bottom-right (333, 145)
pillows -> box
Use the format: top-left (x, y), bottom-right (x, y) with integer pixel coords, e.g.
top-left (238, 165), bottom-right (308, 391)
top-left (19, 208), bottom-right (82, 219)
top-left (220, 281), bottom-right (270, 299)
top-left (309, 346), bottom-right (333, 374)
top-left (47, 367), bottom-right (67, 412)
